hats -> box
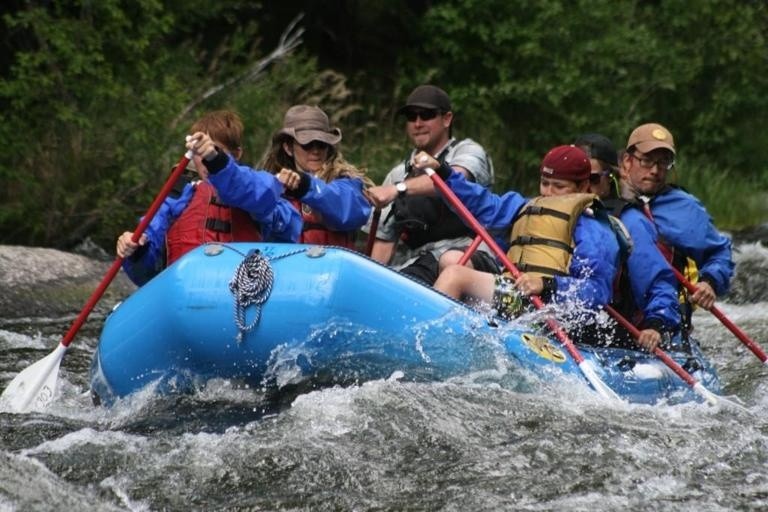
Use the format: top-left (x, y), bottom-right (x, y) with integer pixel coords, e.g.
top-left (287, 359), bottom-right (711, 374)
top-left (574, 132), bottom-right (620, 168)
top-left (396, 84), bottom-right (452, 117)
top-left (625, 122), bottom-right (676, 161)
top-left (539, 144), bottom-right (592, 180)
top-left (272, 103), bottom-right (343, 153)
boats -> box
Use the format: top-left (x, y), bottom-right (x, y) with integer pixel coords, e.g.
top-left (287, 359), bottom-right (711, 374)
top-left (79, 237), bottom-right (715, 409)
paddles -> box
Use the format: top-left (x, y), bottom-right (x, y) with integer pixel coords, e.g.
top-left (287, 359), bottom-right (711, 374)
top-left (423, 168), bottom-right (619, 400)
top-left (0, 148), bottom-right (199, 412)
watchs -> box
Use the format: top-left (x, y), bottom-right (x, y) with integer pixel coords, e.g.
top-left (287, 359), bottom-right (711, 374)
top-left (394, 182), bottom-right (407, 199)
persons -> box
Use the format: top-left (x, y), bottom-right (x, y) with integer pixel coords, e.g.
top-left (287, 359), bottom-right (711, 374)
top-left (254, 105), bottom-right (371, 250)
top-left (621, 122), bottom-right (733, 353)
top-left (412, 144), bottom-right (637, 350)
top-left (574, 132), bottom-right (682, 352)
top-left (360, 85), bottom-right (502, 287)
top-left (116, 110), bottom-right (305, 288)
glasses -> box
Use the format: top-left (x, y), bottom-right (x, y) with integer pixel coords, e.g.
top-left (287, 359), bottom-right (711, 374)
top-left (589, 171), bottom-right (609, 187)
top-left (628, 153), bottom-right (674, 171)
top-left (403, 108), bottom-right (439, 123)
